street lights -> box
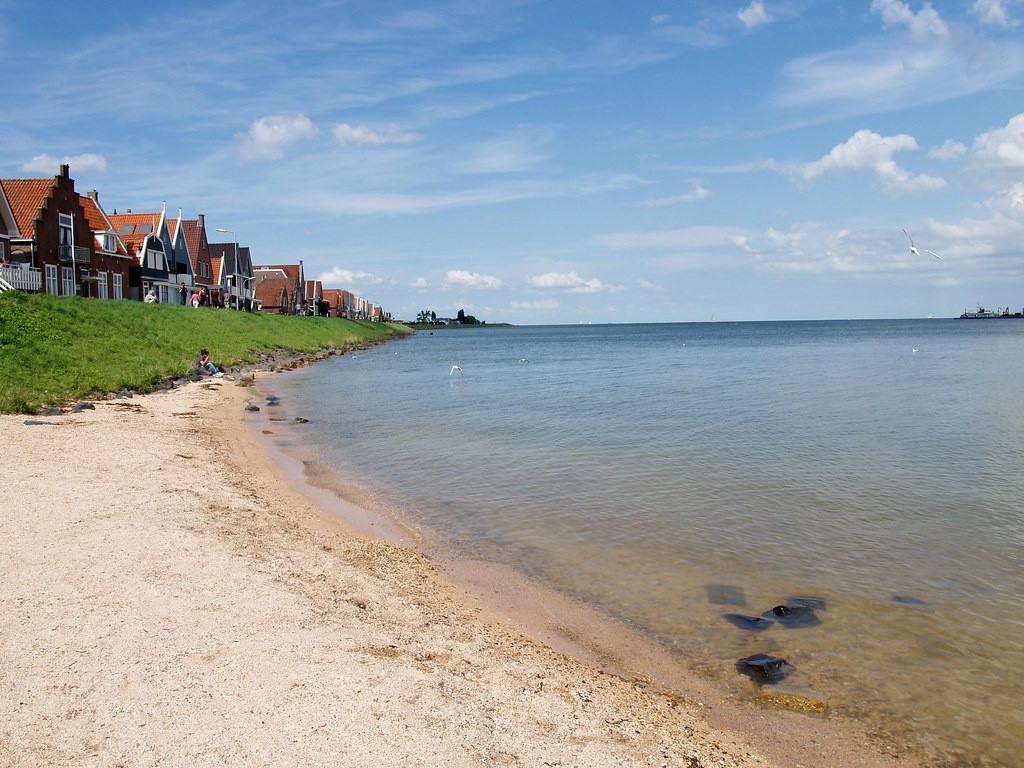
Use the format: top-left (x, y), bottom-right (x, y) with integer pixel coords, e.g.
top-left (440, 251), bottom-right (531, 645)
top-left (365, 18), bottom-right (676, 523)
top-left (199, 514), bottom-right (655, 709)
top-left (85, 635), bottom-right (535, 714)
top-left (214, 228), bottom-right (240, 311)
top-left (243, 275), bottom-right (256, 298)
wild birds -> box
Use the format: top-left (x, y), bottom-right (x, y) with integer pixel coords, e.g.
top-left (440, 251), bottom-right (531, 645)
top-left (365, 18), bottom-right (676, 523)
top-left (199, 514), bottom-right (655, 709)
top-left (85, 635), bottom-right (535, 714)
top-left (449, 365), bottom-right (464, 375)
top-left (912, 348), bottom-right (919, 352)
top-left (901, 228), bottom-right (943, 262)
top-left (519, 359), bottom-right (530, 363)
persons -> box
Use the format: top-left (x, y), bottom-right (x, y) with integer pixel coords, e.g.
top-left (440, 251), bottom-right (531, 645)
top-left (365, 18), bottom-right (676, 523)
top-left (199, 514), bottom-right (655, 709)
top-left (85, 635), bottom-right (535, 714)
top-left (242, 303), bottom-right (245, 311)
top-left (179, 283), bottom-right (188, 305)
top-left (144, 290), bottom-right (157, 303)
top-left (189, 291), bottom-right (201, 308)
top-left (295, 301), bottom-right (301, 313)
top-left (196, 349), bottom-right (224, 378)
top-left (199, 286), bottom-right (208, 307)
top-left (258, 303), bottom-right (263, 311)
top-left (211, 288), bottom-right (219, 306)
top-left (224, 291), bottom-right (232, 308)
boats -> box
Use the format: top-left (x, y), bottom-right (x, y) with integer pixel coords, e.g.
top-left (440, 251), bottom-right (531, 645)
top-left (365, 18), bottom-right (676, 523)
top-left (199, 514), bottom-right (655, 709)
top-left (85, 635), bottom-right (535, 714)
top-left (953, 302), bottom-right (1024, 320)
